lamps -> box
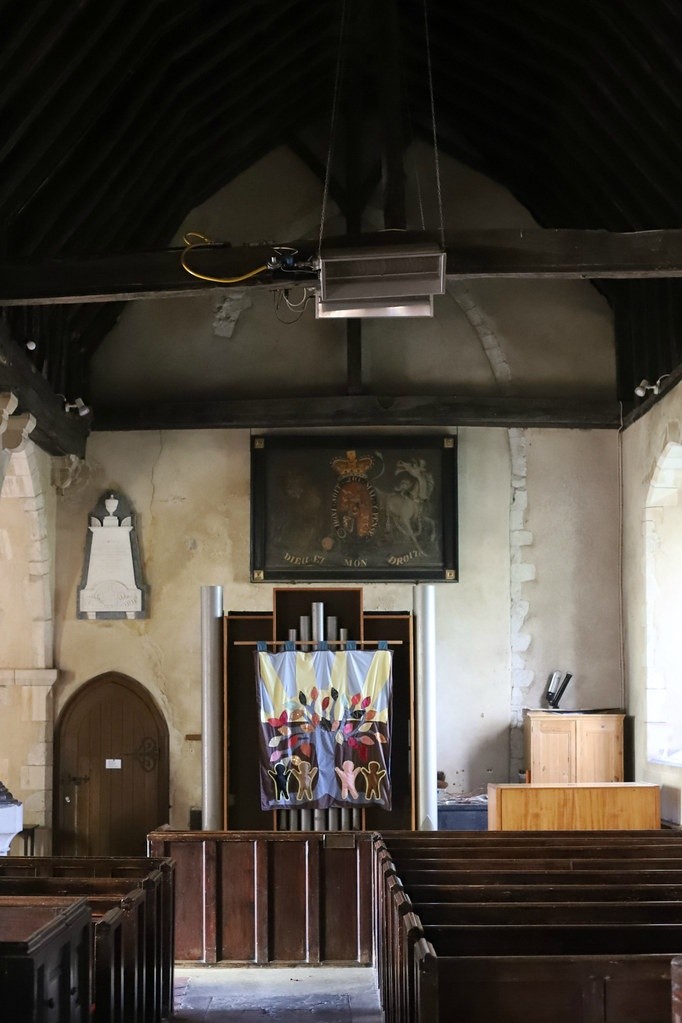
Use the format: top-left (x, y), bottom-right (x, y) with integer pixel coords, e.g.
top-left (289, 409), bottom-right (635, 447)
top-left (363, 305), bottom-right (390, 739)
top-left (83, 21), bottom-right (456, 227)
top-left (65, 397), bottom-right (89, 416)
top-left (635, 379), bottom-right (661, 397)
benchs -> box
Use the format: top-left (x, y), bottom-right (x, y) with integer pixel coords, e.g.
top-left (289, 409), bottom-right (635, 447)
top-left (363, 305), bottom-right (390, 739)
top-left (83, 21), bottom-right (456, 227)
top-left (371, 831), bottom-right (682, 1023)
top-left (0, 854), bottom-right (175, 1023)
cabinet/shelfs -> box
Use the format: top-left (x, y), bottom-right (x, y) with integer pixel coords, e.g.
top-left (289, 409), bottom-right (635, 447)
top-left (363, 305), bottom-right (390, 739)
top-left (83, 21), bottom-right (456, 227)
top-left (522, 709), bottom-right (627, 783)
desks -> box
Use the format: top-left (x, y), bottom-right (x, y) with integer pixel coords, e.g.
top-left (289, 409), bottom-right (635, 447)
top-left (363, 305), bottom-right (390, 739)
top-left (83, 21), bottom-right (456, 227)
top-left (487, 782), bottom-right (661, 830)
